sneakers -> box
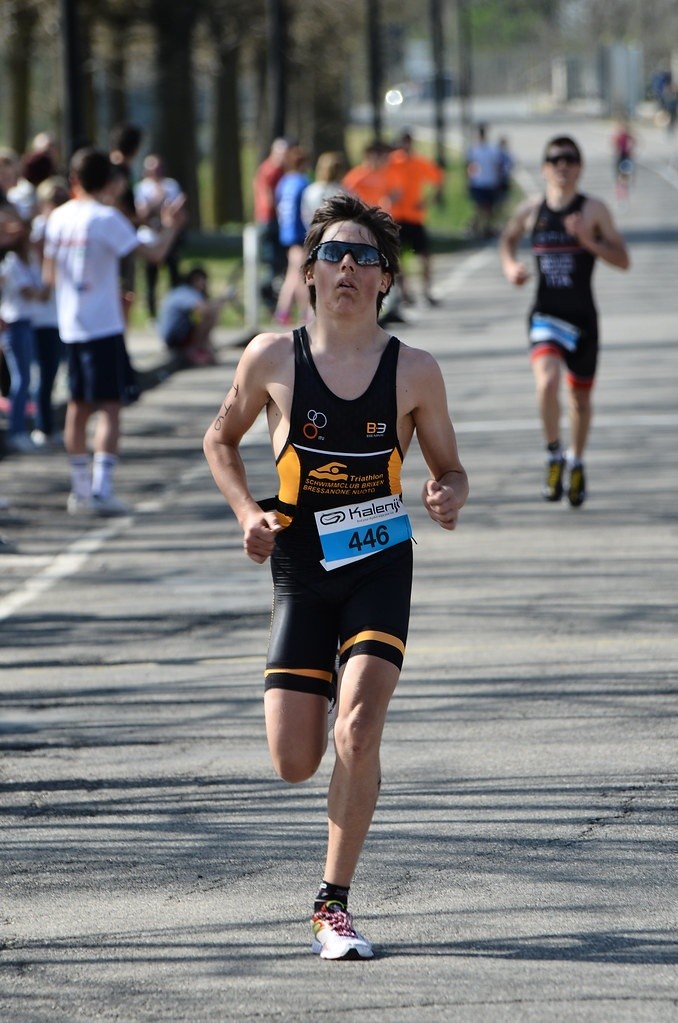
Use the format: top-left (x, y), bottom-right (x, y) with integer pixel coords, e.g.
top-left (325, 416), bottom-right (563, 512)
top-left (325, 654), bottom-right (340, 730)
top-left (311, 905), bottom-right (374, 961)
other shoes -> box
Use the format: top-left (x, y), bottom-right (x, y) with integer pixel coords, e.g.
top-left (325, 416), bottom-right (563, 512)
top-left (564, 450), bottom-right (585, 507)
top-left (67, 492), bottom-right (133, 517)
top-left (542, 455), bottom-right (566, 500)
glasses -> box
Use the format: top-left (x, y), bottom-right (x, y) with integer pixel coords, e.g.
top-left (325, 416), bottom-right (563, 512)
top-left (307, 240), bottom-right (390, 268)
top-left (546, 154), bottom-right (583, 168)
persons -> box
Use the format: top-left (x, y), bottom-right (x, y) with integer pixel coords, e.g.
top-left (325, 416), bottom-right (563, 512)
top-left (205, 194), bottom-right (470, 957)
top-left (465, 123), bottom-right (514, 237)
top-left (0, 123), bottom-right (143, 453)
top-left (500, 137), bottom-right (631, 507)
top-left (132, 155), bottom-right (180, 319)
top-left (612, 123), bottom-right (636, 196)
top-left (249, 134), bottom-right (445, 329)
top-left (156, 268), bottom-right (220, 363)
top-left (41, 148), bottom-right (187, 516)
